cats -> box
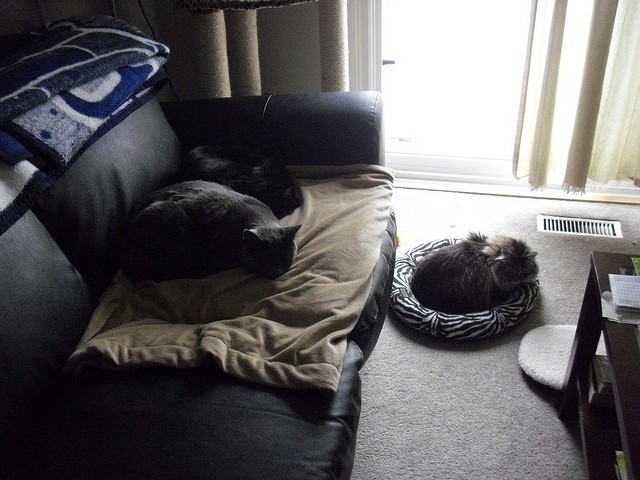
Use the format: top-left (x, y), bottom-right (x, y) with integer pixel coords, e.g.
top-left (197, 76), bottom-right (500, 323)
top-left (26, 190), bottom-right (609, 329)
top-left (412, 231), bottom-right (540, 314)
top-left (172, 143), bottom-right (302, 220)
top-left (121, 181), bottom-right (302, 284)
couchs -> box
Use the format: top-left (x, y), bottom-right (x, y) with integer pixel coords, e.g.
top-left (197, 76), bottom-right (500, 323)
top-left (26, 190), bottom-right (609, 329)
top-left (1, 92), bottom-right (396, 479)
